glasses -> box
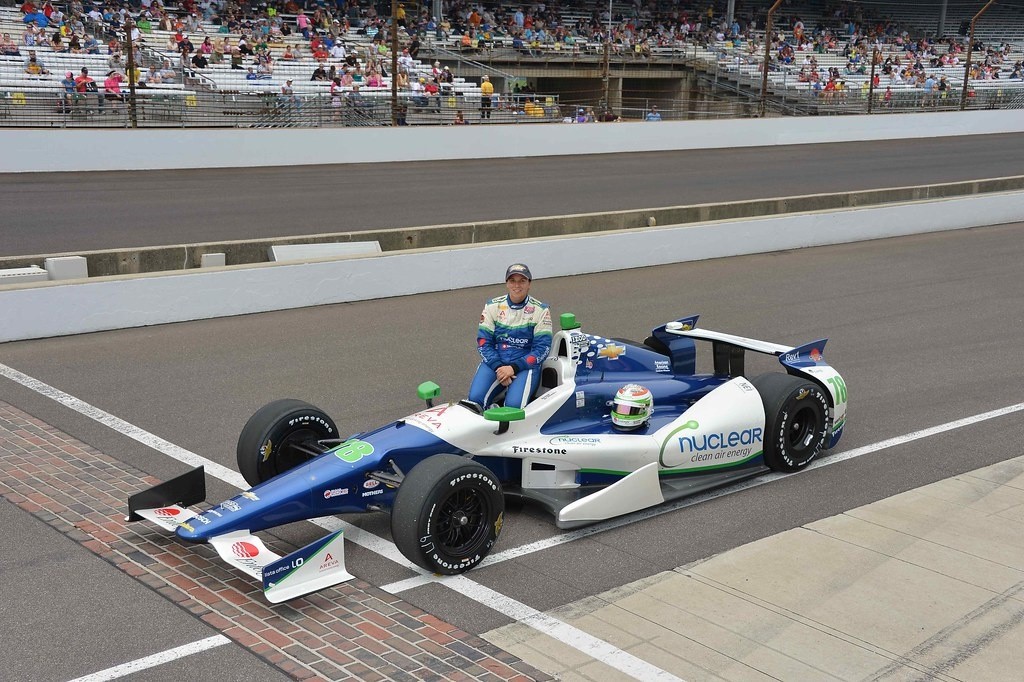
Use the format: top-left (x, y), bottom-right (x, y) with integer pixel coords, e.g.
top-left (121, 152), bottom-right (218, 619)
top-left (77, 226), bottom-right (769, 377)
top-left (26, 26), bottom-right (32, 28)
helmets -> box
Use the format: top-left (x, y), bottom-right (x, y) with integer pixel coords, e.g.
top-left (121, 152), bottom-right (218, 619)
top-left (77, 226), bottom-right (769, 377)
top-left (610, 385), bottom-right (653, 430)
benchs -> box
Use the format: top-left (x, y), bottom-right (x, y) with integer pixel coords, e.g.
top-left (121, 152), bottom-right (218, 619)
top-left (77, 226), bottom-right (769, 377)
top-left (0, 0), bottom-right (1023, 110)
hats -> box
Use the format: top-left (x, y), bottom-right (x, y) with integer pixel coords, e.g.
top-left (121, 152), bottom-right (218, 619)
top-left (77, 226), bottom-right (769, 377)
top-left (482, 75), bottom-right (489, 81)
top-left (506, 263), bottom-right (531, 282)
top-left (258, 56), bottom-right (266, 62)
top-left (105, 69), bottom-right (115, 76)
top-left (419, 78), bottom-right (425, 84)
top-left (82, 67), bottom-right (89, 73)
top-left (287, 77), bottom-right (292, 81)
top-left (65, 71), bottom-right (73, 77)
top-left (215, 47), bottom-right (225, 54)
top-left (578, 109), bottom-right (584, 114)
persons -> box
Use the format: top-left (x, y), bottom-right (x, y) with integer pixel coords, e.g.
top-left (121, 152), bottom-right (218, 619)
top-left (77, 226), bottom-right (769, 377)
top-left (646, 105), bottom-right (664, 121)
top-left (408, 34), bottom-right (420, 59)
top-left (480, 73), bottom-right (494, 117)
top-left (873, 74), bottom-right (879, 87)
top-left (397, 69), bottom-right (412, 91)
top-left (861, 80), bottom-right (870, 99)
top-left (297, 8), bottom-right (310, 35)
top-left (137, 16), bottom-right (153, 34)
top-left (468, 263), bottom-right (553, 409)
top-left (991, 66), bottom-right (1001, 79)
top-left (52, 33), bottom-right (66, 58)
top-left (514, 5), bottom-right (524, 28)
top-left (470, 9), bottom-right (482, 27)
top-left (104, 68), bottom-right (125, 114)
top-left (68, 36), bottom-right (83, 54)
top-left (75, 67), bottom-right (106, 115)
top-left (61, 71), bottom-right (79, 114)
top-left (83, 34), bottom-right (99, 54)
top-left (395, 3), bottom-right (408, 27)
top-left (0, 0), bottom-right (1024, 125)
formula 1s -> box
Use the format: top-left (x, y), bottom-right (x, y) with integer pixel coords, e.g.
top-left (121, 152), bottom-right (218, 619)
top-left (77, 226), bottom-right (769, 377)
top-left (124, 313), bottom-right (848, 604)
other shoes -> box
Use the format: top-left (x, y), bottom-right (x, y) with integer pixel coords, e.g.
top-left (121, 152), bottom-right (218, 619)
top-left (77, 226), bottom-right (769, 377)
top-left (86, 110), bottom-right (94, 114)
top-left (99, 110), bottom-right (106, 116)
